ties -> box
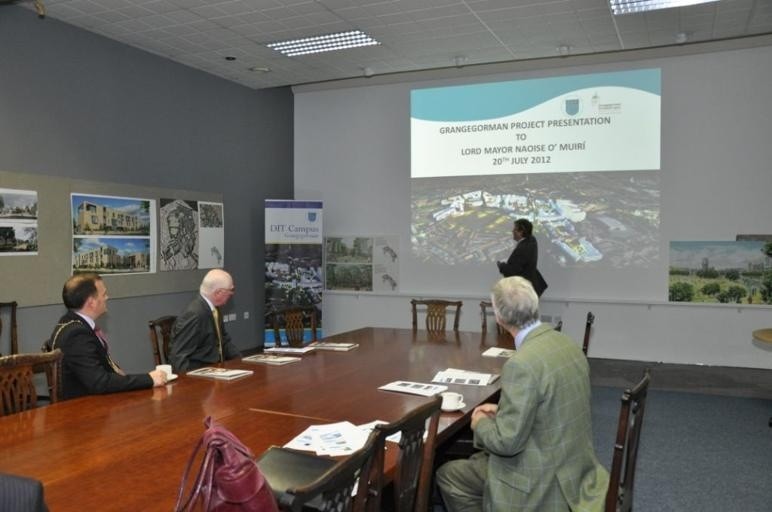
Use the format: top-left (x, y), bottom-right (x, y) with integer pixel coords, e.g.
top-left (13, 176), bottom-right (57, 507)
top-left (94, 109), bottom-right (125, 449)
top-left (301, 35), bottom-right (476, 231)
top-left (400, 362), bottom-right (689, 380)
top-left (213, 309), bottom-right (223, 362)
top-left (95, 327), bottom-right (124, 375)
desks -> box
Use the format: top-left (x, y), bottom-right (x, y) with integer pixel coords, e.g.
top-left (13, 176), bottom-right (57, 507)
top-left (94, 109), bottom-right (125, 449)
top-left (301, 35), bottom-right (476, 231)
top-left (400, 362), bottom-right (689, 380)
top-left (0, 326), bottom-right (517, 511)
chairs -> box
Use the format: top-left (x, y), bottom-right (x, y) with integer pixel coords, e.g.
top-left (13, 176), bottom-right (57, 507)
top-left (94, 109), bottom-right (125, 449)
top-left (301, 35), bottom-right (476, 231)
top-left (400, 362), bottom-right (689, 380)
top-left (605, 366), bottom-right (650, 512)
top-left (409, 298), bottom-right (464, 330)
top-left (272, 306), bottom-right (316, 347)
top-left (0, 348), bottom-right (65, 418)
top-left (479, 301), bottom-right (510, 334)
top-left (148, 315), bottom-right (177, 364)
top-left (369, 394), bottom-right (443, 512)
top-left (583, 313), bottom-right (594, 355)
top-left (278, 429), bottom-right (382, 512)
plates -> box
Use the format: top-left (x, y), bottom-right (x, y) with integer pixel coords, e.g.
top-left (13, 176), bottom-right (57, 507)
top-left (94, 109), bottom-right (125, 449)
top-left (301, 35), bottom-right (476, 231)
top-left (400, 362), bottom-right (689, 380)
top-left (439, 402), bottom-right (465, 412)
top-left (166, 374), bottom-right (178, 381)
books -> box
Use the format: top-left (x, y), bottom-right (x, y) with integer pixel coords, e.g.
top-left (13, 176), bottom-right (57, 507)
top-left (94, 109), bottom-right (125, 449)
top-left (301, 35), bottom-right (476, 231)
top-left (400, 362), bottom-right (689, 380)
top-left (308, 342), bottom-right (359, 351)
top-left (242, 354), bottom-right (302, 365)
top-left (264, 347), bottom-right (313, 352)
top-left (186, 366), bottom-right (254, 380)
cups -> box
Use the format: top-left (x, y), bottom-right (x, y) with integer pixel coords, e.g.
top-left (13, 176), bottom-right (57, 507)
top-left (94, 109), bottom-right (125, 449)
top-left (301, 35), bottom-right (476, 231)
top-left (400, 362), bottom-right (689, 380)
top-left (156, 364), bottom-right (172, 377)
top-left (441, 392), bottom-right (464, 407)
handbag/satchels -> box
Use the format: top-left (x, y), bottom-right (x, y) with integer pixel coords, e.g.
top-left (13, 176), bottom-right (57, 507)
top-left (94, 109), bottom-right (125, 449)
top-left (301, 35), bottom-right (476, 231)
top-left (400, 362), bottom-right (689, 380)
top-left (174, 416), bottom-right (281, 511)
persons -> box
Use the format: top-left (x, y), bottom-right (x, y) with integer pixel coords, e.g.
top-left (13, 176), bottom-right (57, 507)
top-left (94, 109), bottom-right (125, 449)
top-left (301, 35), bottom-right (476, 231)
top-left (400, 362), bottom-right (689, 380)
top-left (165, 268), bottom-right (243, 373)
top-left (435, 274), bottom-right (609, 511)
top-left (46, 273), bottom-right (168, 400)
top-left (0, 473), bottom-right (49, 512)
top-left (496, 218), bottom-right (548, 297)
